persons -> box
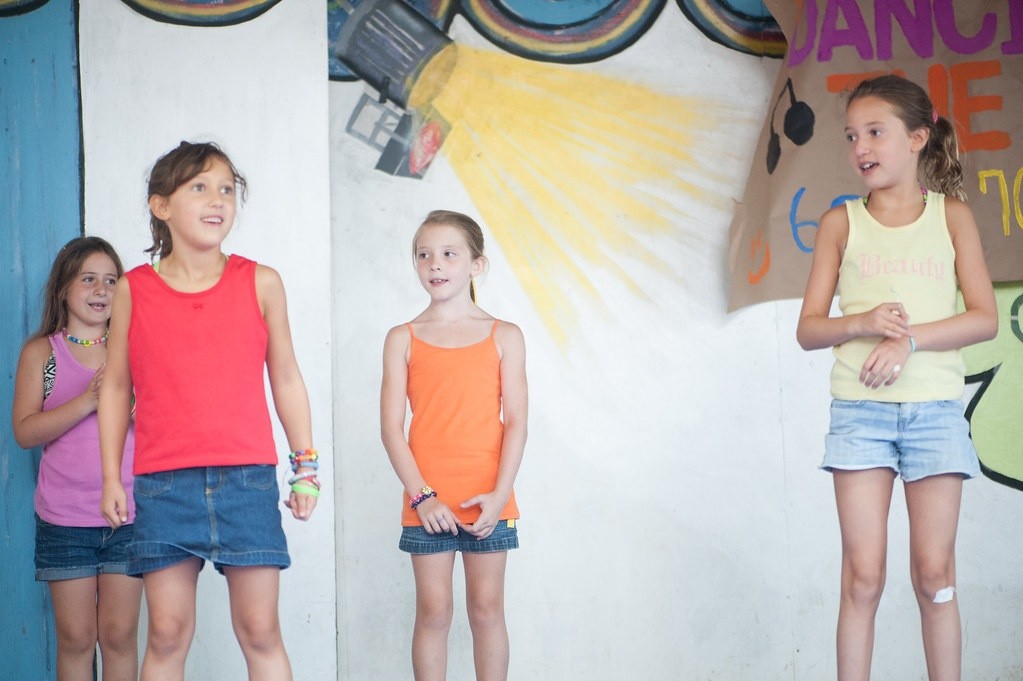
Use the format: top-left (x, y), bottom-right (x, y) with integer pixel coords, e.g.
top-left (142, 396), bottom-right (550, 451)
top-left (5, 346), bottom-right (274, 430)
top-left (10, 237), bottom-right (146, 681)
top-left (380, 211), bottom-right (529, 681)
top-left (797, 76), bottom-right (997, 681)
top-left (98, 140), bottom-right (321, 681)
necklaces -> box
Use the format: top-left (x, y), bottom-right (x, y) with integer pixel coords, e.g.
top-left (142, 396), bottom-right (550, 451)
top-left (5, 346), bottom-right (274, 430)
top-left (860, 183), bottom-right (930, 214)
top-left (61, 325), bottom-right (110, 347)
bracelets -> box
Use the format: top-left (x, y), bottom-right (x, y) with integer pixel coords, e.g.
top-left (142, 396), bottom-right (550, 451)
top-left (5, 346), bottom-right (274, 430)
top-left (905, 327), bottom-right (916, 353)
top-left (409, 486), bottom-right (438, 512)
top-left (289, 451), bottom-right (320, 499)
